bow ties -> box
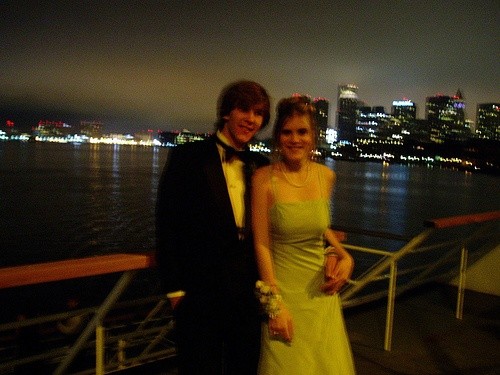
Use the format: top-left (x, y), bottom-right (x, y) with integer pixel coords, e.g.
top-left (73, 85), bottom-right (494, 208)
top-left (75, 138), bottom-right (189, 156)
top-left (214, 138), bottom-right (251, 163)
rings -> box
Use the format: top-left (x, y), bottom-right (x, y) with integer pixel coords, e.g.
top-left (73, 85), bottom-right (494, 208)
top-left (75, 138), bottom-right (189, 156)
top-left (274, 330), bottom-right (280, 336)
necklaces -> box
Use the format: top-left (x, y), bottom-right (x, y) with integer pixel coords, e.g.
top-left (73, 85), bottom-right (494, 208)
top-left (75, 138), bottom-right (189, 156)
top-left (278, 159), bottom-right (313, 188)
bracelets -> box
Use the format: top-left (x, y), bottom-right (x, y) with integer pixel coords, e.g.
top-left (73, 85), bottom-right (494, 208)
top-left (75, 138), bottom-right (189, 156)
top-left (254, 278), bottom-right (285, 320)
top-left (326, 253), bottom-right (340, 259)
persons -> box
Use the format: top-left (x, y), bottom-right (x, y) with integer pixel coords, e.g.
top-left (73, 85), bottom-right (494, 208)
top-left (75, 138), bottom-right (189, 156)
top-left (152, 79), bottom-right (346, 374)
top-left (248, 95), bottom-right (356, 374)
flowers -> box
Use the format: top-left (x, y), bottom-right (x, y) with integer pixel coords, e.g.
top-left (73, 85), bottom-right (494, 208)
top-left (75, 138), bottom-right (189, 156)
top-left (256, 280), bottom-right (282, 320)
